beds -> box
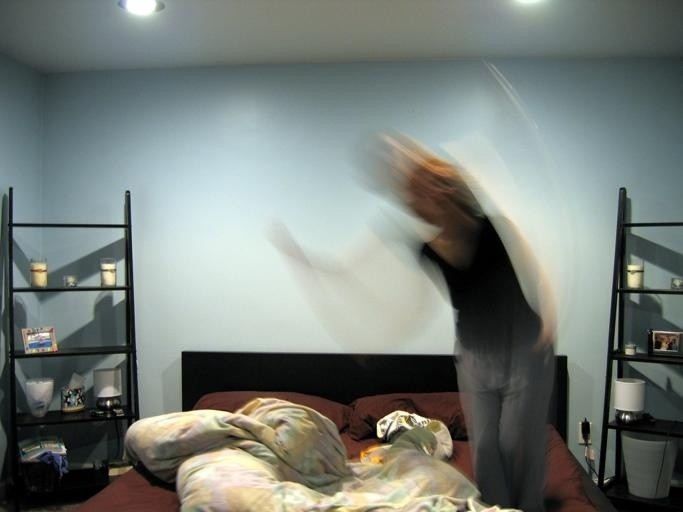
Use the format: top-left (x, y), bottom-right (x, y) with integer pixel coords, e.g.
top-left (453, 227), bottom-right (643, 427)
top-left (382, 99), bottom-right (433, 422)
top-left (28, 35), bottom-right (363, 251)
top-left (69, 350), bottom-right (595, 512)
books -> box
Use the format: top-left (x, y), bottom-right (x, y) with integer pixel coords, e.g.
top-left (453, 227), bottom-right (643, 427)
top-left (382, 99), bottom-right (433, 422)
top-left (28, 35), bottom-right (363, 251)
top-left (16, 433), bottom-right (65, 461)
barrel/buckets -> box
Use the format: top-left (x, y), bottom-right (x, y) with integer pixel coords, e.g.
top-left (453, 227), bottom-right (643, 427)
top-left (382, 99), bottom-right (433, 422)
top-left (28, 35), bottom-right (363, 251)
top-left (620, 431), bottom-right (679, 499)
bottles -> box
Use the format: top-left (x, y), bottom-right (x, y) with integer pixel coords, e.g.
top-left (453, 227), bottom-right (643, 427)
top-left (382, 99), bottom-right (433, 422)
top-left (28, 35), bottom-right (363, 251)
top-left (100, 259), bottom-right (116, 288)
top-left (627, 264), bottom-right (644, 289)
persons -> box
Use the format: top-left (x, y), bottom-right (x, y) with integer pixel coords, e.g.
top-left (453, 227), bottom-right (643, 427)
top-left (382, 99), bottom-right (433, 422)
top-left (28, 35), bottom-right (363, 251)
top-left (655, 335), bottom-right (676, 349)
top-left (400, 158), bottom-right (560, 511)
top-left (62, 387), bottom-right (84, 406)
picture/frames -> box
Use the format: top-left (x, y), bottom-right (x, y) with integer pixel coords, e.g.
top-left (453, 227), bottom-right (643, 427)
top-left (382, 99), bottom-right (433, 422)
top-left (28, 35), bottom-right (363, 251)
top-left (651, 331), bottom-right (681, 355)
top-left (19, 326), bottom-right (57, 355)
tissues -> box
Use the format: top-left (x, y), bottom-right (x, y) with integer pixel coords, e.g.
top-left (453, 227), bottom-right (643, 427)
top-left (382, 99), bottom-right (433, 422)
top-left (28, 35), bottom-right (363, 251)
top-left (59, 372), bottom-right (87, 412)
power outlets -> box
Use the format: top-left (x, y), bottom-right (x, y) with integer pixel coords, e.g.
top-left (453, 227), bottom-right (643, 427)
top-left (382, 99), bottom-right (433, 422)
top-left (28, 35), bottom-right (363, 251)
top-left (577, 421), bottom-right (592, 446)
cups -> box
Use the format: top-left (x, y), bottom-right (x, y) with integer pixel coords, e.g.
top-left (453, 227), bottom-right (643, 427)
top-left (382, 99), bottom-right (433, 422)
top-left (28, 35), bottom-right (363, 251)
top-left (63, 273), bottom-right (79, 288)
top-left (625, 344), bottom-right (636, 355)
top-left (26, 377), bottom-right (54, 417)
top-left (670, 278), bottom-right (683, 290)
top-left (60, 384), bottom-right (87, 413)
top-left (30, 257), bottom-right (48, 287)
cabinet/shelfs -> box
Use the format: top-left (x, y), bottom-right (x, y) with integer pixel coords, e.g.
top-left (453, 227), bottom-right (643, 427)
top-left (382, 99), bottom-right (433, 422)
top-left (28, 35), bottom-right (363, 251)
top-left (596, 187), bottom-right (682, 512)
top-left (7, 186), bottom-right (140, 512)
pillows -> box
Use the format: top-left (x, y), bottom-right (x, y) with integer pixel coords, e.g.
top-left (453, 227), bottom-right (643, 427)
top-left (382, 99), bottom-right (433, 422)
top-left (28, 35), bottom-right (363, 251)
top-left (192, 391), bottom-right (467, 443)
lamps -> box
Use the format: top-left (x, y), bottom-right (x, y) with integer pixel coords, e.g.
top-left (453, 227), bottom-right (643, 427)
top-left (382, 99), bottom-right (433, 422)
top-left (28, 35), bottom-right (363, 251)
top-left (90, 368), bottom-right (124, 418)
top-left (612, 378), bottom-right (645, 423)
